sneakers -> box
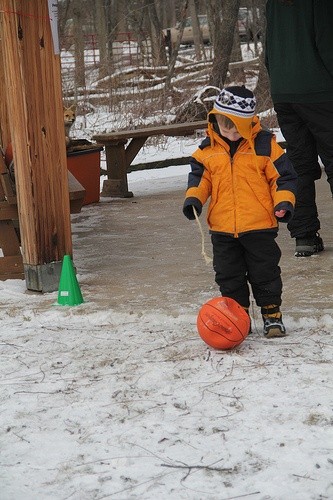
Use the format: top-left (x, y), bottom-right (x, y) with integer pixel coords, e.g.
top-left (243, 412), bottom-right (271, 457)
top-left (261, 304), bottom-right (287, 338)
top-left (294, 231), bottom-right (324, 257)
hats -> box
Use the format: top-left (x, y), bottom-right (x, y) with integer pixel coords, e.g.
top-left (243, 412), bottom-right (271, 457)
top-left (208, 84), bottom-right (261, 178)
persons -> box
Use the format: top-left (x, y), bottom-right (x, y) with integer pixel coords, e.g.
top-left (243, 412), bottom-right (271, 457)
top-left (264, 0), bottom-right (333, 255)
top-left (183, 86), bottom-right (297, 339)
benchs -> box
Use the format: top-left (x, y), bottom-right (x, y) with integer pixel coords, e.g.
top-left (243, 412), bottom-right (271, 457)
top-left (93, 119), bottom-right (210, 198)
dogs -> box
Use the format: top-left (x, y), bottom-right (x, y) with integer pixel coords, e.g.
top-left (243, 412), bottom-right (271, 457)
top-left (63, 105), bottom-right (77, 146)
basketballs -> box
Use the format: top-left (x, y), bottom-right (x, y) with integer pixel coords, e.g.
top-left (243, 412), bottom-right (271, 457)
top-left (196, 296), bottom-right (250, 351)
